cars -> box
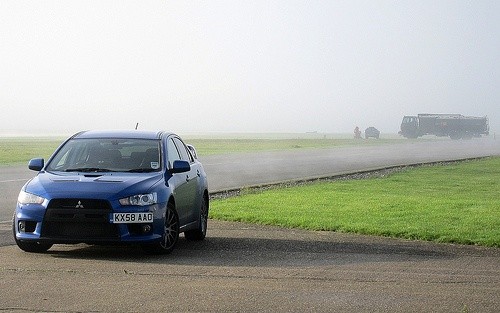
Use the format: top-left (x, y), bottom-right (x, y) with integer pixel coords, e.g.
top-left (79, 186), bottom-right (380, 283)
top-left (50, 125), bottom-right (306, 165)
top-left (12, 122), bottom-right (211, 254)
top-left (365, 126), bottom-right (381, 139)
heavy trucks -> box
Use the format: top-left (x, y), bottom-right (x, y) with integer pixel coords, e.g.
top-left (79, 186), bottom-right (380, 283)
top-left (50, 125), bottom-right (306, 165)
top-left (398, 113), bottom-right (489, 138)
